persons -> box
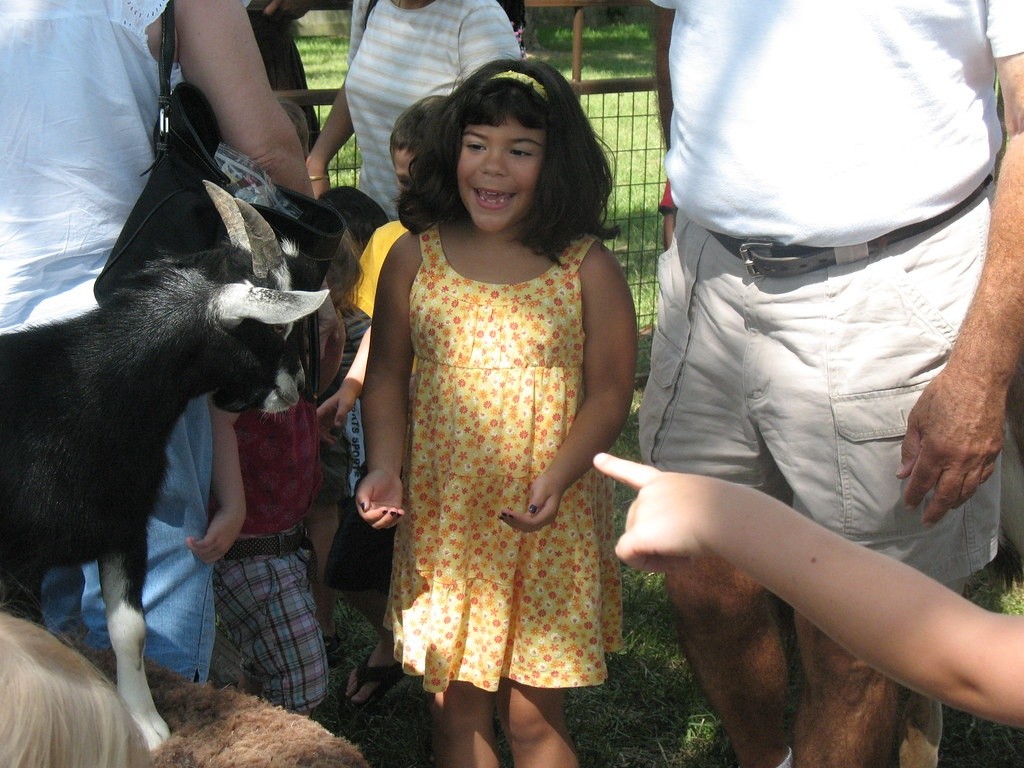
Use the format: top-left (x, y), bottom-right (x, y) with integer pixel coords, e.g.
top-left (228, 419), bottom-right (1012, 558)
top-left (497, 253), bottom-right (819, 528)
top-left (642, 0), bottom-right (1023, 768)
top-left (592, 452), bottom-right (1024, 728)
top-left (356, 63), bottom-right (638, 768)
top-left (311, 96), bottom-right (449, 704)
top-left (0, 1), bottom-right (329, 768)
top-left (307, 0), bottom-right (525, 218)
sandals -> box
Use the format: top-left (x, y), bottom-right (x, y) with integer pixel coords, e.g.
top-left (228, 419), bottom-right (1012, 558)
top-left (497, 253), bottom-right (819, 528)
top-left (343, 652), bottom-right (404, 708)
top-left (320, 631), bottom-right (348, 669)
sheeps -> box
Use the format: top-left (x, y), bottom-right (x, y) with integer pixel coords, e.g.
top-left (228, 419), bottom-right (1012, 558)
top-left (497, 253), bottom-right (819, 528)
top-left (0, 177), bottom-right (334, 755)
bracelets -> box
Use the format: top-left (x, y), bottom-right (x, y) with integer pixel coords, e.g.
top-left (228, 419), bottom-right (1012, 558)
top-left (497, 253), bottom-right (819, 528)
top-left (309, 175), bottom-right (330, 183)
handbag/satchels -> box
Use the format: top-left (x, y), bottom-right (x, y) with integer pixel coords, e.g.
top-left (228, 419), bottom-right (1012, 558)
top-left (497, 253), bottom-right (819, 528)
top-left (93, 0), bottom-right (345, 306)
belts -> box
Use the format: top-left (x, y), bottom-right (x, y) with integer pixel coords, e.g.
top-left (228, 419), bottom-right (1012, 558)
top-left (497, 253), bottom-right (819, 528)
top-left (223, 524), bottom-right (317, 584)
top-left (708, 175), bottom-right (992, 277)
